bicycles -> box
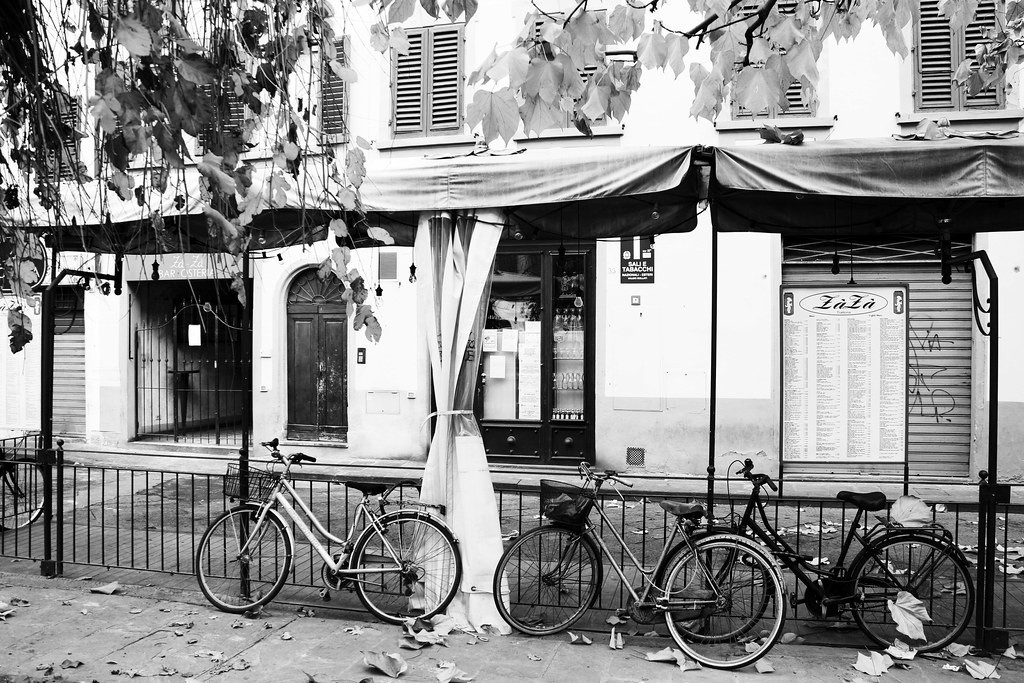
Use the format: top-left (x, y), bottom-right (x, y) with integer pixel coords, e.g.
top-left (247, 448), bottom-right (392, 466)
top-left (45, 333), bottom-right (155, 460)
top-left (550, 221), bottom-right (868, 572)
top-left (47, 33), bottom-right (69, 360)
top-left (491, 461), bottom-right (788, 670)
top-left (193, 435), bottom-right (465, 626)
top-left (0, 451), bottom-right (44, 531)
top-left (662, 458), bottom-right (976, 657)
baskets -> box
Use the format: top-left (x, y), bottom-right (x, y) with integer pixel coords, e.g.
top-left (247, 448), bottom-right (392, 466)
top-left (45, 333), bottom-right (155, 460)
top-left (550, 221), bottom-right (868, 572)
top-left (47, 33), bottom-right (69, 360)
top-left (224, 463), bottom-right (276, 502)
top-left (540, 479), bottom-right (594, 525)
